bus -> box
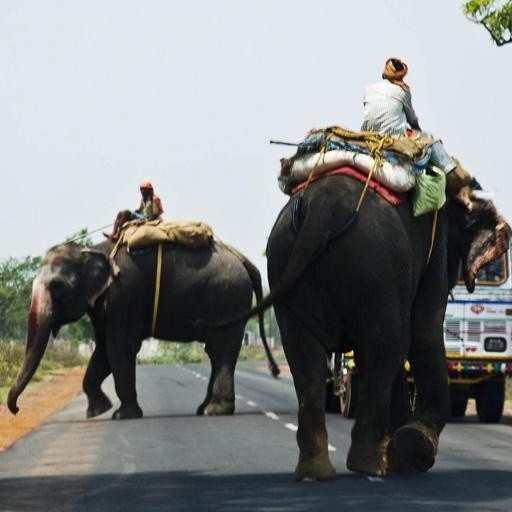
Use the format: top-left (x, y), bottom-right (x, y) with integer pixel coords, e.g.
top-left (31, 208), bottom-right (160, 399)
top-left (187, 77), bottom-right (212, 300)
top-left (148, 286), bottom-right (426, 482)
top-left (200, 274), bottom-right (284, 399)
top-left (325, 217), bottom-right (512, 420)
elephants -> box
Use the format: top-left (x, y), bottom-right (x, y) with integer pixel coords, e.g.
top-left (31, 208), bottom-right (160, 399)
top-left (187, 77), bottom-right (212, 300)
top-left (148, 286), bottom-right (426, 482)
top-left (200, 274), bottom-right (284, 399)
top-left (187, 154), bottom-right (512, 483)
top-left (7, 239), bottom-right (281, 421)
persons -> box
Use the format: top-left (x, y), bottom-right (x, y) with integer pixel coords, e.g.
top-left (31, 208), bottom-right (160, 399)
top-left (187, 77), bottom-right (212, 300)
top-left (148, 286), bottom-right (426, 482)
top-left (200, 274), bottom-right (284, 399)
top-left (102, 180), bottom-right (164, 242)
top-left (360, 58), bottom-right (423, 137)
